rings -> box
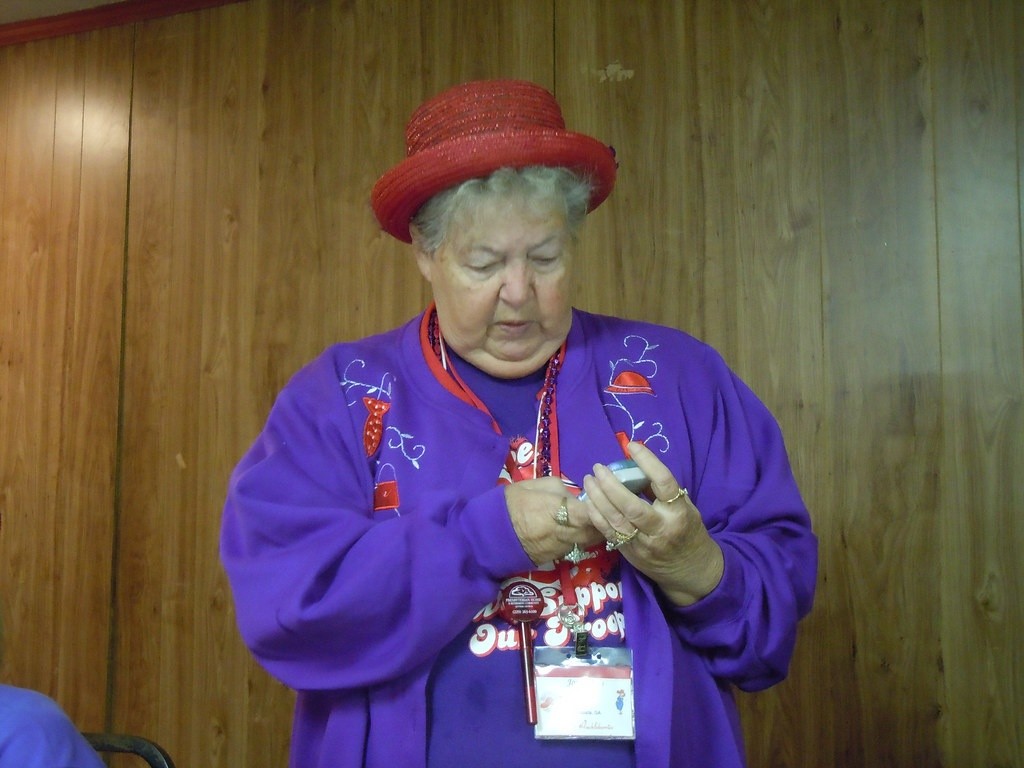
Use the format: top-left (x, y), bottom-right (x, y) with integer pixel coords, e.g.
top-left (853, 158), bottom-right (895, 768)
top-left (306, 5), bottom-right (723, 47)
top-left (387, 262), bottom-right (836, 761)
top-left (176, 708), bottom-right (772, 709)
top-left (616, 528), bottom-right (638, 543)
top-left (666, 486), bottom-right (688, 503)
top-left (556, 496), bottom-right (569, 525)
top-left (564, 542), bottom-right (591, 565)
top-left (603, 541), bottom-right (624, 552)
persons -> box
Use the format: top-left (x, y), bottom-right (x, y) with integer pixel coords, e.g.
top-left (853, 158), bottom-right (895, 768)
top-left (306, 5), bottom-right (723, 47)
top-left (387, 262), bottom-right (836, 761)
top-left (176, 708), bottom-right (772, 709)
top-left (0, 683), bottom-right (109, 768)
top-left (220, 79), bottom-right (821, 768)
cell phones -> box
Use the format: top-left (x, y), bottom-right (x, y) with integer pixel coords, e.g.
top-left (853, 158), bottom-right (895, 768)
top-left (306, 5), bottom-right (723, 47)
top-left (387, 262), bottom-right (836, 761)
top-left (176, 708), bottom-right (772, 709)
top-left (577, 460), bottom-right (652, 508)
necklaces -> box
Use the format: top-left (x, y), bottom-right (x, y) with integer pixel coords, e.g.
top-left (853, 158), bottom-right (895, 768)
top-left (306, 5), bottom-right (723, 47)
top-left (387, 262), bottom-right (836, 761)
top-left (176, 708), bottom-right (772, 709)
top-left (428, 310), bottom-right (560, 725)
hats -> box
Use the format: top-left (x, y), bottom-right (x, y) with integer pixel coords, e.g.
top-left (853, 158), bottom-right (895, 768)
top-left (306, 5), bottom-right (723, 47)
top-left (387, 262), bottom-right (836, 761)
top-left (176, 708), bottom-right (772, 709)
top-left (370, 81), bottom-right (618, 243)
